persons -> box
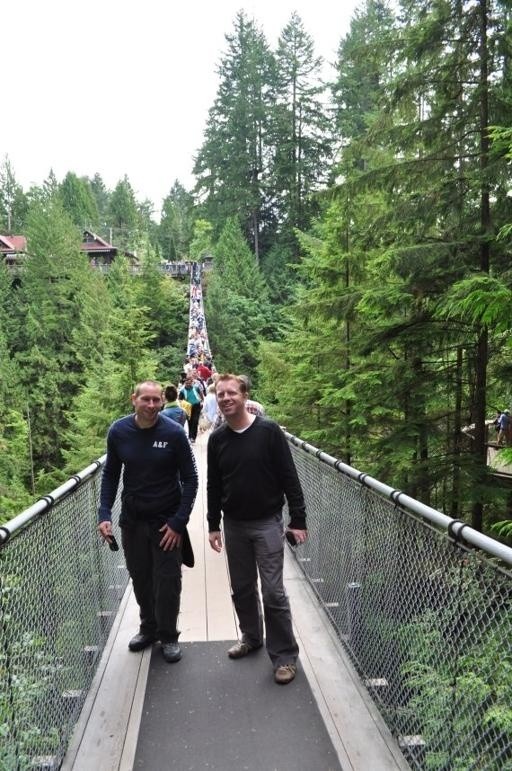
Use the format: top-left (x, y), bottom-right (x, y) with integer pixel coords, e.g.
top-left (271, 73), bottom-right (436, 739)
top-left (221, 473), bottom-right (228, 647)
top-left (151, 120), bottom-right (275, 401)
top-left (91, 256), bottom-right (96, 272)
top-left (97, 380), bottom-right (199, 663)
top-left (159, 259), bottom-right (265, 444)
top-left (207, 373), bottom-right (308, 684)
top-left (494, 408), bottom-right (512, 450)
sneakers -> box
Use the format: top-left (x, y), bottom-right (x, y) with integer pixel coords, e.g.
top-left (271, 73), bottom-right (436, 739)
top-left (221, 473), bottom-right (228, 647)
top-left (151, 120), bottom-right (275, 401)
top-left (160, 635), bottom-right (183, 662)
top-left (274, 662), bottom-right (298, 684)
top-left (128, 629), bottom-right (156, 651)
top-left (226, 634), bottom-right (263, 658)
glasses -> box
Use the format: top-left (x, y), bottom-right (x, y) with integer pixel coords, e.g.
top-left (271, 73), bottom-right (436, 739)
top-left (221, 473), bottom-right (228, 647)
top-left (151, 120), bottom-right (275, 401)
top-left (102, 535), bottom-right (119, 551)
